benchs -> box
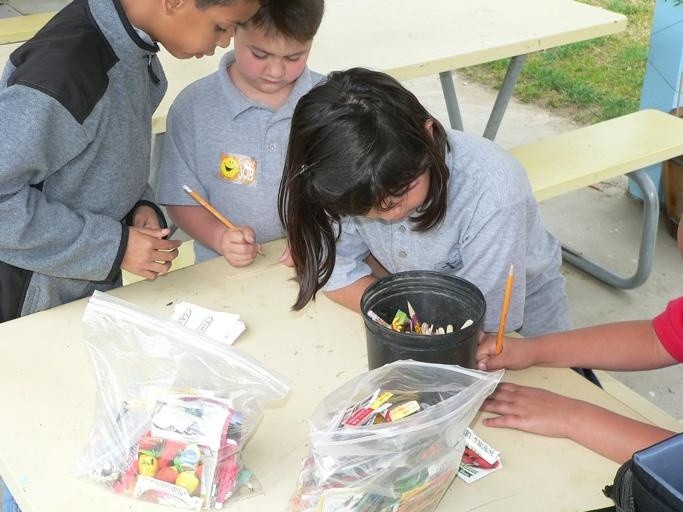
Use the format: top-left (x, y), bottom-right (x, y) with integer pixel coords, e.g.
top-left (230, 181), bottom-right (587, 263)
top-left (587, 364), bottom-right (680, 438)
top-left (512, 109), bottom-right (682, 291)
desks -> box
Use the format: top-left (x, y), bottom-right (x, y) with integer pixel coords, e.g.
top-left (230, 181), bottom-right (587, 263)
top-left (0, 230), bottom-right (660, 512)
top-left (1, 1), bottom-right (626, 144)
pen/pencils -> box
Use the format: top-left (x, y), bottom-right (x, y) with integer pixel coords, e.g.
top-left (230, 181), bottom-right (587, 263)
top-left (495, 264), bottom-right (514, 354)
top-left (182, 186), bottom-right (266, 256)
top-left (367, 300), bottom-right (474, 335)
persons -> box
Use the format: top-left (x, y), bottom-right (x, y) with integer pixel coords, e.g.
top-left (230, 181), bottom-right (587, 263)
top-left (278, 67), bottom-right (586, 379)
top-left (477, 296), bottom-right (683, 466)
top-left (156, 0), bottom-right (328, 267)
top-left (0, 0), bottom-right (265, 323)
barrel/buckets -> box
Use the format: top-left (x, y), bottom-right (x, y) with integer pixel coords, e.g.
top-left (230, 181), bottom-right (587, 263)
top-left (663, 106), bottom-right (683, 242)
top-left (358, 267), bottom-right (487, 407)
